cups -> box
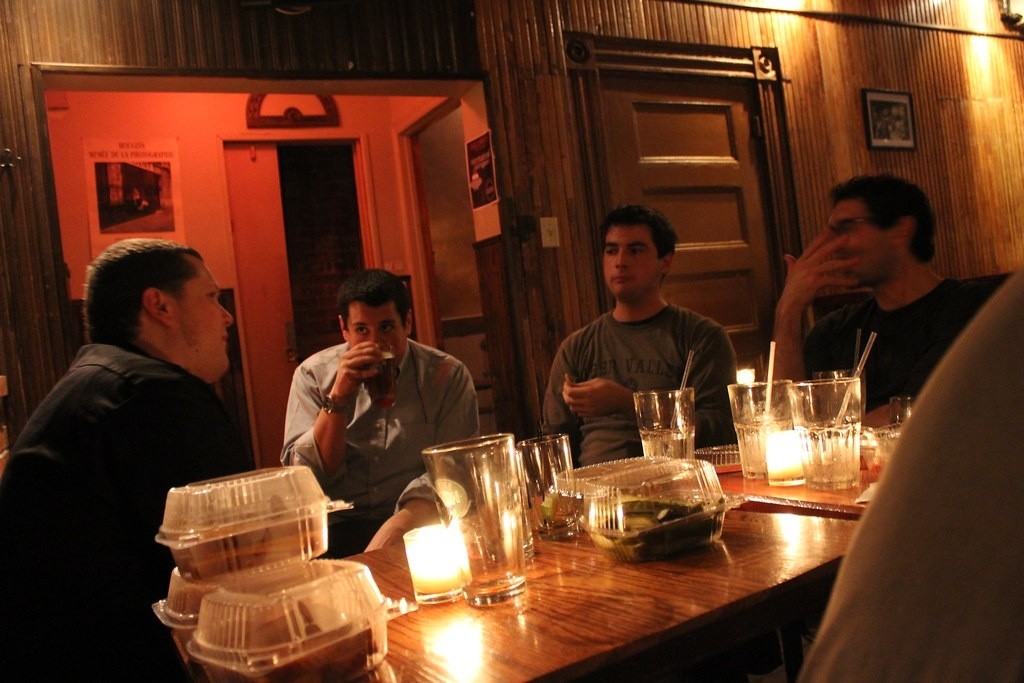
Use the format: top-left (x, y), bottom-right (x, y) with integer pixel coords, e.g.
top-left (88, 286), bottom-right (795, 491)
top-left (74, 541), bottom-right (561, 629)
top-left (813, 369), bottom-right (866, 425)
top-left (466, 450), bottom-right (535, 564)
top-left (727, 380), bottom-right (792, 479)
top-left (518, 435), bottom-right (580, 541)
top-left (422, 433), bottom-right (527, 608)
top-left (362, 343), bottom-right (395, 390)
top-left (890, 396), bottom-right (917, 430)
top-left (633, 388), bottom-right (695, 459)
top-left (403, 525), bottom-right (463, 605)
top-left (787, 377), bottom-right (862, 490)
top-left (765, 432), bottom-right (806, 487)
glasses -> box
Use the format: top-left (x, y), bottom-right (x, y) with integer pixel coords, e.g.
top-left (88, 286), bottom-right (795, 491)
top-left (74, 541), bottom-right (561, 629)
top-left (834, 217), bottom-right (870, 234)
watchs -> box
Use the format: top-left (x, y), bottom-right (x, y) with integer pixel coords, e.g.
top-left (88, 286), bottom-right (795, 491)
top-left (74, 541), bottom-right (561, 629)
top-left (319, 393), bottom-right (354, 416)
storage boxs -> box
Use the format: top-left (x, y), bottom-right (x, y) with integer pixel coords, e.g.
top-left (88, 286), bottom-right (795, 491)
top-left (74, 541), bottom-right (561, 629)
top-left (551, 455), bottom-right (745, 562)
top-left (151, 465), bottom-right (419, 682)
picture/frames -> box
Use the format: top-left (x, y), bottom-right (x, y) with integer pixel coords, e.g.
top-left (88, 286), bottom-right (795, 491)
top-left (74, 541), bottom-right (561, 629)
top-left (861, 87), bottom-right (919, 152)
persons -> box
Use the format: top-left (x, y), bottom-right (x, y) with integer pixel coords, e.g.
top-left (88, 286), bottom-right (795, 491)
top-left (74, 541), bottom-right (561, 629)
top-left (1, 236), bottom-right (252, 679)
top-left (279, 268), bottom-right (481, 550)
top-left (766, 174), bottom-right (1015, 428)
top-left (545, 206), bottom-right (739, 463)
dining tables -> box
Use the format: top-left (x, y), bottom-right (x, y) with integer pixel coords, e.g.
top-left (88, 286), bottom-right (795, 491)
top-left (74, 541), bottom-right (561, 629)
top-left (334, 509), bottom-right (861, 683)
top-left (715, 466), bottom-right (890, 682)
